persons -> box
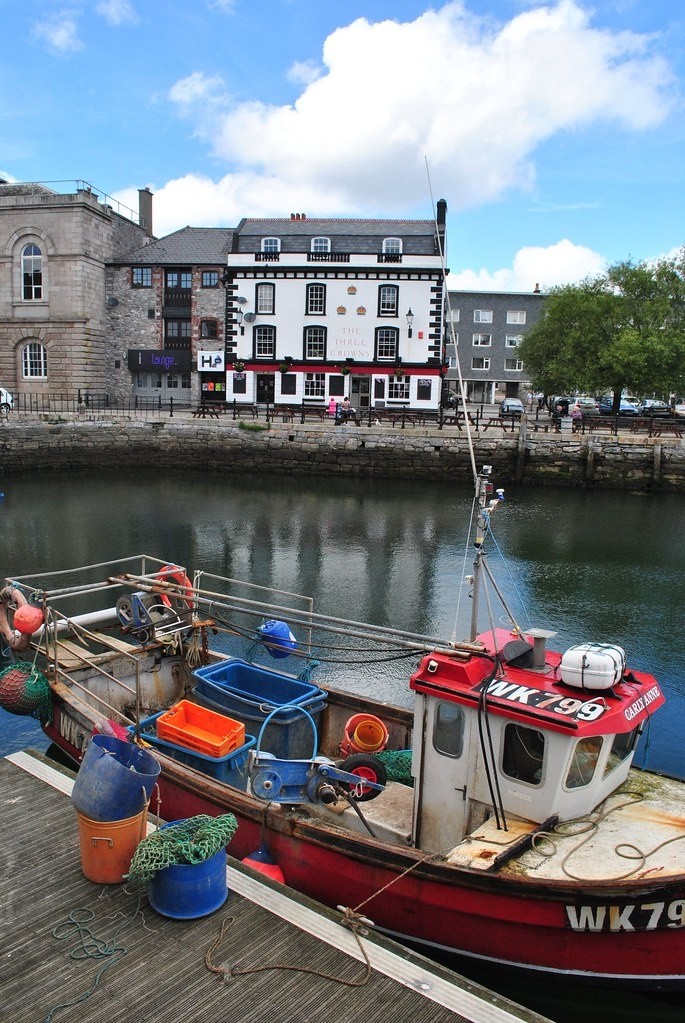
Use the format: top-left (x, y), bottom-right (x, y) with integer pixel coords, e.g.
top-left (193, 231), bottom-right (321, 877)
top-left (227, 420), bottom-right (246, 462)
top-left (551, 406), bottom-right (562, 433)
top-left (329, 398), bottom-right (336, 414)
top-left (571, 406), bottom-right (581, 433)
top-left (341, 397), bottom-right (351, 412)
top-left (450, 396), bottom-right (454, 407)
top-left (527, 389), bottom-right (535, 411)
top-left (536, 392), bottom-right (544, 410)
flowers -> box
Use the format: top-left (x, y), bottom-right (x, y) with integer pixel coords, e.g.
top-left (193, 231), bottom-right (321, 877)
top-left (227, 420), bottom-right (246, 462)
top-left (232, 361), bottom-right (245, 370)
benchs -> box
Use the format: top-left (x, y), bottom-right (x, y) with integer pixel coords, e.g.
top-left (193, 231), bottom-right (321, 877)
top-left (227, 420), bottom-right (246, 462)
top-left (192, 398), bottom-right (685, 437)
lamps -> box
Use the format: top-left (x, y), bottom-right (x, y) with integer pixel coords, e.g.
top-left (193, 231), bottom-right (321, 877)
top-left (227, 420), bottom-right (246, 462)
top-left (346, 358), bottom-right (354, 364)
top-left (406, 306), bottom-right (415, 340)
top-left (433, 358), bottom-right (440, 364)
top-left (236, 306), bottom-right (244, 335)
top-left (285, 356), bottom-right (293, 363)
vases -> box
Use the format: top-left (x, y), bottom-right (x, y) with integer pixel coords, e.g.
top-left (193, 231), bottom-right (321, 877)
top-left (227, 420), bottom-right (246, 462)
top-left (236, 368), bottom-right (243, 372)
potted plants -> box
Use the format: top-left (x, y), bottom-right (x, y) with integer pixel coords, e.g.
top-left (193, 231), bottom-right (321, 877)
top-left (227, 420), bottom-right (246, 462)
top-left (394, 370), bottom-right (404, 377)
top-left (279, 364), bottom-right (288, 373)
top-left (341, 367), bottom-right (350, 375)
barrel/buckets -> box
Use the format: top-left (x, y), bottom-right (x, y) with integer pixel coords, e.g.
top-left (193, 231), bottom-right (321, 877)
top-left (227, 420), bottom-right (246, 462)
top-left (341, 713), bottom-right (389, 760)
top-left (153, 816), bottom-right (228, 920)
top-left (71, 734), bottom-right (162, 821)
top-left (259, 619), bottom-right (297, 659)
top-left (353, 720), bottom-right (385, 751)
top-left (74, 801), bottom-right (151, 884)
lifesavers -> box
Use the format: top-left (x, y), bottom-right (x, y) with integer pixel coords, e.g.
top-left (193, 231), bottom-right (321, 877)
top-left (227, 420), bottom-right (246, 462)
top-left (0, 587), bottom-right (30, 650)
top-left (157, 565), bottom-right (197, 620)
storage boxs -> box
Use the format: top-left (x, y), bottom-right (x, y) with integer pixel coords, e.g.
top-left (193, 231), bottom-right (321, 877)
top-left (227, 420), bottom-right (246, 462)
top-left (127, 658), bottom-right (327, 793)
